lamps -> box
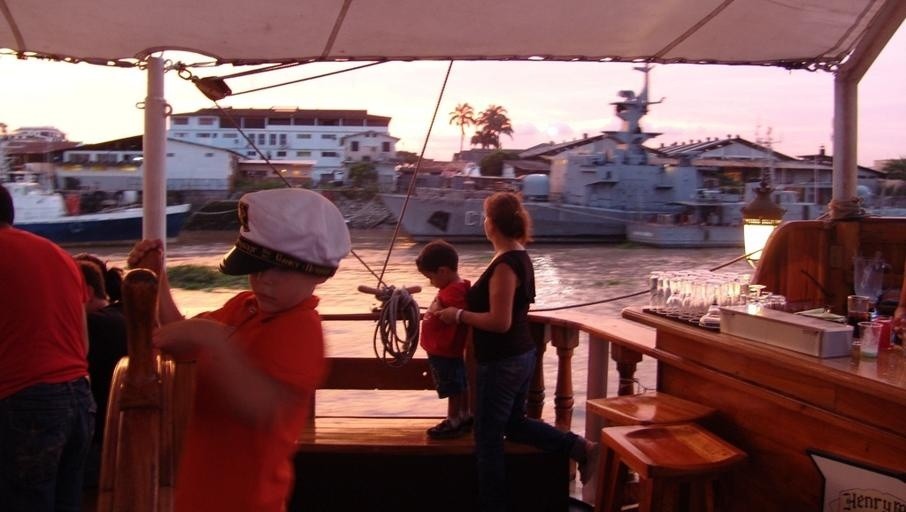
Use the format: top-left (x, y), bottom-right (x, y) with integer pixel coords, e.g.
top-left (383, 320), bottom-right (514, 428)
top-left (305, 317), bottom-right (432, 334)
top-left (742, 179), bottom-right (787, 271)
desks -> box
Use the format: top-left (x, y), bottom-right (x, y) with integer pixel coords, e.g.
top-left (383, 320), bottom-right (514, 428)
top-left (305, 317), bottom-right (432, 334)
top-left (622, 300), bottom-right (906, 511)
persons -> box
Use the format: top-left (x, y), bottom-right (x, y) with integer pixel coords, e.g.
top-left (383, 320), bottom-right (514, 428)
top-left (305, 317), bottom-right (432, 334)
top-left (0, 187), bottom-right (127, 512)
top-left (433, 194), bottom-right (600, 512)
top-left (417, 240), bottom-right (474, 438)
top-left (127, 189), bottom-right (351, 512)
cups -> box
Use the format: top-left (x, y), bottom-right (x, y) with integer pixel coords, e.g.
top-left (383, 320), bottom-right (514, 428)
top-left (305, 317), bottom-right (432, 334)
top-left (847, 256), bottom-right (893, 358)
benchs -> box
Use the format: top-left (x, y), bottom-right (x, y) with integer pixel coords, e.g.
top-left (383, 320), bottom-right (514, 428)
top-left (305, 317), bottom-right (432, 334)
top-left (282, 418), bottom-right (547, 455)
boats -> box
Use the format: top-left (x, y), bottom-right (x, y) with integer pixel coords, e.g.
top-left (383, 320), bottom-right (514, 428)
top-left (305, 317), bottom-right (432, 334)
top-left (627, 187), bottom-right (823, 249)
top-left (377, 188), bottom-right (638, 245)
top-left (4, 182), bottom-right (191, 248)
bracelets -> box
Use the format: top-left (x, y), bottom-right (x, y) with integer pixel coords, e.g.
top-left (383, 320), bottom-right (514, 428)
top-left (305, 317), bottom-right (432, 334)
top-left (456, 308), bottom-right (464, 325)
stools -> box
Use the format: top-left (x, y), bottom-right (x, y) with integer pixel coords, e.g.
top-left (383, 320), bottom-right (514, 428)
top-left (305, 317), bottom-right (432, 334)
top-left (583, 392), bottom-right (720, 512)
top-left (595, 422), bottom-right (748, 512)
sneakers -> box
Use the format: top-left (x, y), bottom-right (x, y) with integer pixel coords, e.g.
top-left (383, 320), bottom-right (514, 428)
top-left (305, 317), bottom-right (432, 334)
top-left (578, 442), bottom-right (599, 484)
top-left (427, 417), bottom-right (474, 439)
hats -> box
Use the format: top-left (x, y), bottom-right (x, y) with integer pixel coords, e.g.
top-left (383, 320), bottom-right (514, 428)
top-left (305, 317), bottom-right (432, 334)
top-left (218, 188), bottom-right (351, 276)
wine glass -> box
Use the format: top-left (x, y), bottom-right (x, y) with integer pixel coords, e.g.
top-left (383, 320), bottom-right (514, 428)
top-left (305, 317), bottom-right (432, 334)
top-left (650, 270), bottom-right (787, 326)
